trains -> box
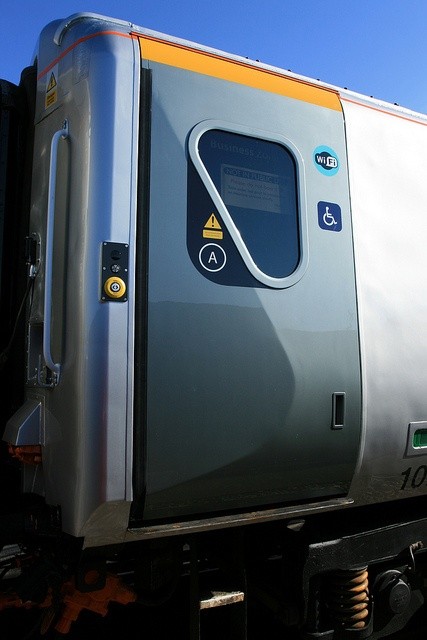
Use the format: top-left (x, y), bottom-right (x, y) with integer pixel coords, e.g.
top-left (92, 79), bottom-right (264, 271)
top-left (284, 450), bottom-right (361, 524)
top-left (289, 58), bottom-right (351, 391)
top-left (0, 12), bottom-right (426, 639)
